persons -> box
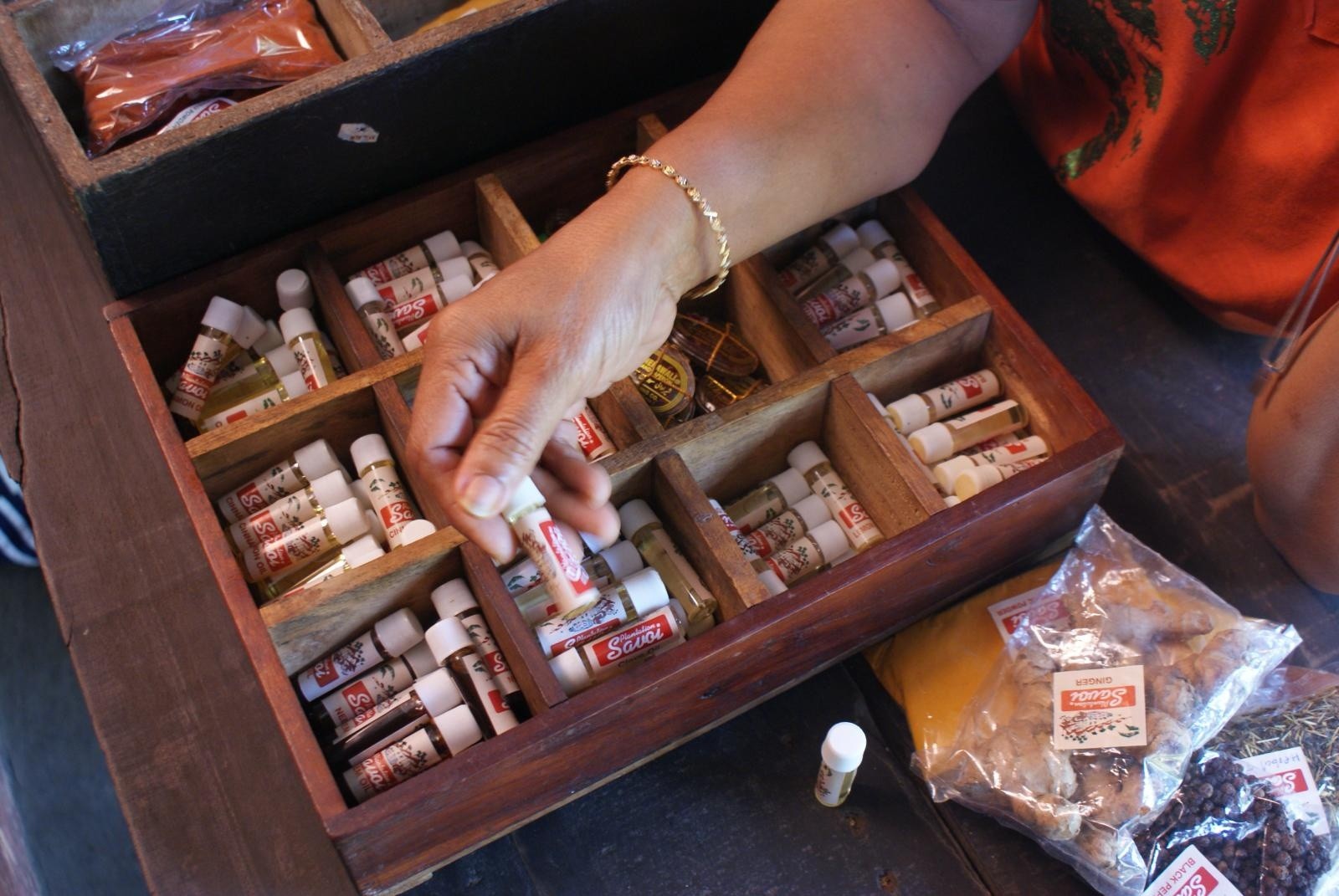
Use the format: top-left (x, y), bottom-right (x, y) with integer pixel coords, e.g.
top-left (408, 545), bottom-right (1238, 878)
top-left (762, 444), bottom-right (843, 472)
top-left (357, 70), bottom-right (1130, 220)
top-left (401, 0), bottom-right (1339, 602)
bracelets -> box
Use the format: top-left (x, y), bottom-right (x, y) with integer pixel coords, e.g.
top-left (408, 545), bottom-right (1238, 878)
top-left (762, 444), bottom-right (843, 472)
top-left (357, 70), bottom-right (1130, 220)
top-left (607, 155), bottom-right (733, 301)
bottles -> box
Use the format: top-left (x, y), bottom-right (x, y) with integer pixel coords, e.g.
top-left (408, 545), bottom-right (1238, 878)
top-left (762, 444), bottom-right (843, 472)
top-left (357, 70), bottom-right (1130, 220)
top-left (164, 218), bottom-right (1053, 809)
